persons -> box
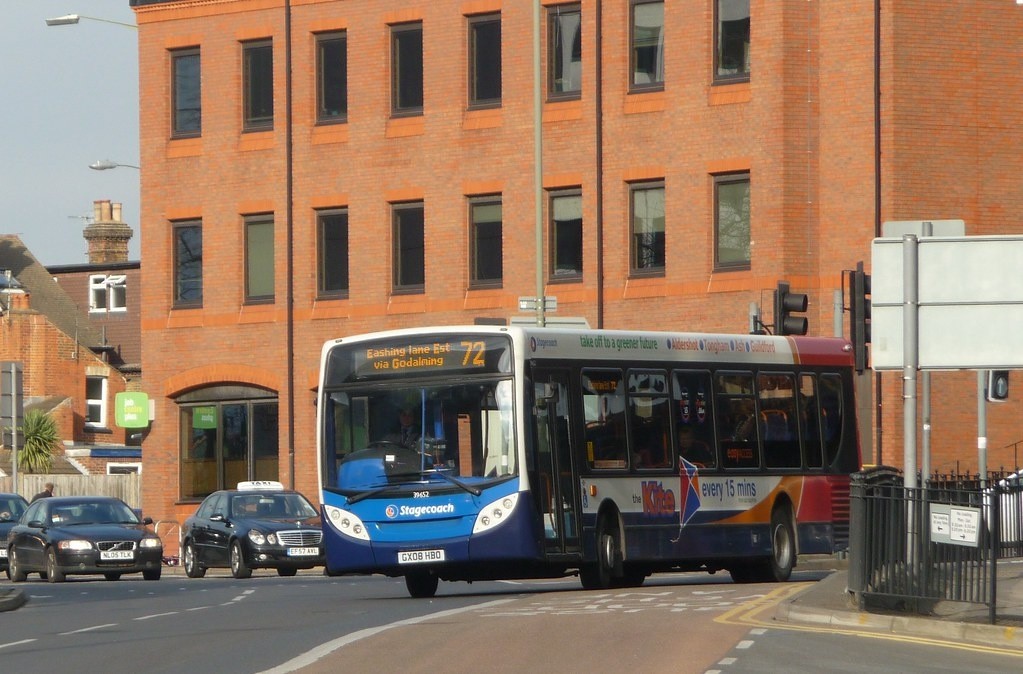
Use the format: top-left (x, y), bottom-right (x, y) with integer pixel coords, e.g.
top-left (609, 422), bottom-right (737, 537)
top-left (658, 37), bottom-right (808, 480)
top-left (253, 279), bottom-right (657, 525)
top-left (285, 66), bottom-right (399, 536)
top-left (30, 483), bottom-right (54, 504)
top-left (381, 405), bottom-right (422, 449)
top-left (0, 505), bottom-right (14, 520)
top-left (680, 426), bottom-right (712, 465)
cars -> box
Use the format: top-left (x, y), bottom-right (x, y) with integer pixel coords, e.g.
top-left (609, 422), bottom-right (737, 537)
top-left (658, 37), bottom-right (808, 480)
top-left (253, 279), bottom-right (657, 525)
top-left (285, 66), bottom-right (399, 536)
top-left (0, 493), bottom-right (34, 579)
top-left (180, 480), bottom-right (327, 582)
top-left (8, 496), bottom-right (162, 583)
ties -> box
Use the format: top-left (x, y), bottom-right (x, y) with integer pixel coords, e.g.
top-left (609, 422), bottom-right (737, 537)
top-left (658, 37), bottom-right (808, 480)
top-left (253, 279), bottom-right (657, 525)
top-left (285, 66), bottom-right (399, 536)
top-left (402, 428), bottom-right (408, 443)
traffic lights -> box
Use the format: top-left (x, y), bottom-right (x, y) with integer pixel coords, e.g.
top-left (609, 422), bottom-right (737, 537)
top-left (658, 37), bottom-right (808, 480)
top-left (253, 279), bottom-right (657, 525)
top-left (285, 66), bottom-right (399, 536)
top-left (988, 370), bottom-right (1013, 402)
top-left (851, 258), bottom-right (872, 375)
top-left (769, 281), bottom-right (810, 337)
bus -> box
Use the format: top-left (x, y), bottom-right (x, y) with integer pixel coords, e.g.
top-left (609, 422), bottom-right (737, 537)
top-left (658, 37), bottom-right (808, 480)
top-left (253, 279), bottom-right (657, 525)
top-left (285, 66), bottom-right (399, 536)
top-left (317, 326), bottom-right (864, 590)
